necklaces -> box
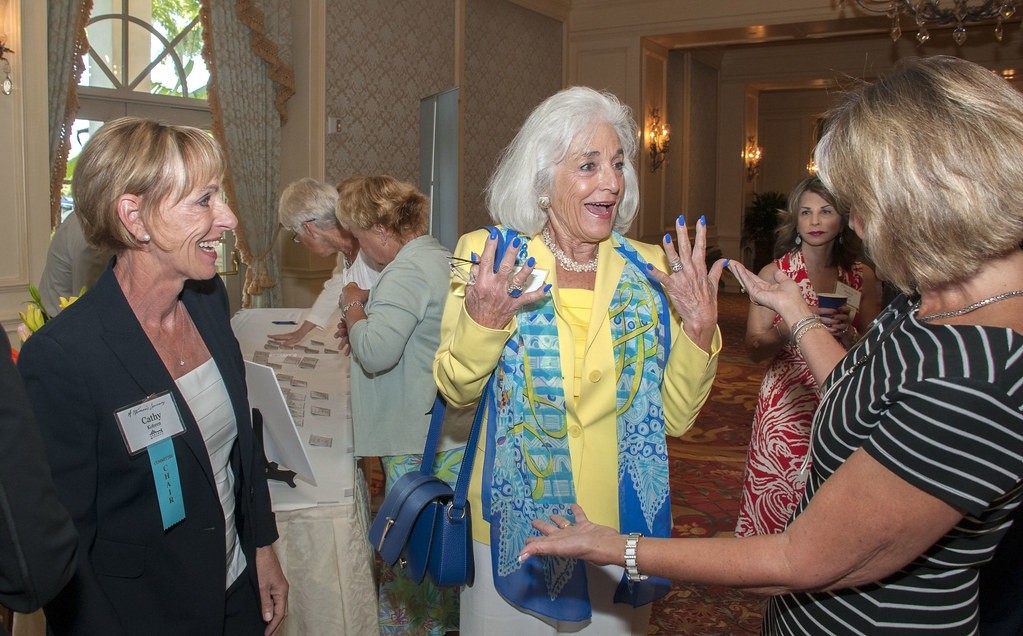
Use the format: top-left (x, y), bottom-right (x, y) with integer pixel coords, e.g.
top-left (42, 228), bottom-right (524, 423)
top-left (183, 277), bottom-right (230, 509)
top-left (343, 256), bottom-right (352, 269)
top-left (911, 290), bottom-right (1022, 323)
top-left (541, 227), bottom-right (599, 273)
top-left (142, 300), bottom-right (185, 365)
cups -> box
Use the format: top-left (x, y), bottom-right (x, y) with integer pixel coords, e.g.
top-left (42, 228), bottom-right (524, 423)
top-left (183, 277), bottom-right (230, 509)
top-left (818, 293), bottom-right (847, 327)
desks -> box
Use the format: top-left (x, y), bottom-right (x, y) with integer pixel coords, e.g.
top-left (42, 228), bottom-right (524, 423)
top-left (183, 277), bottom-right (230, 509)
top-left (231, 309), bottom-right (369, 636)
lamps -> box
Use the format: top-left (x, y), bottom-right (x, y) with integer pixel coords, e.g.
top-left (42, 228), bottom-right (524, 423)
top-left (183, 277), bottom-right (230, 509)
top-left (831, 0), bottom-right (1023, 45)
top-left (649, 107), bottom-right (669, 173)
top-left (748, 136), bottom-right (762, 179)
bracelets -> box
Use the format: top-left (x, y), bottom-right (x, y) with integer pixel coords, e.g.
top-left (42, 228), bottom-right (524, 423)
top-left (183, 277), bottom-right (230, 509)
top-left (625, 533), bottom-right (652, 582)
top-left (849, 326), bottom-right (858, 345)
top-left (789, 314), bottom-right (829, 358)
top-left (341, 301), bottom-right (364, 318)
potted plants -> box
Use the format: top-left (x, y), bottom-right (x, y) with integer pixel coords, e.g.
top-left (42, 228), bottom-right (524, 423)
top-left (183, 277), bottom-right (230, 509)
top-left (740, 190), bottom-right (789, 272)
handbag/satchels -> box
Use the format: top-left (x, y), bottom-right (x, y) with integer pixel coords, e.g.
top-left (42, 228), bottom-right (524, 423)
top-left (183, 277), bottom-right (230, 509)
top-left (368, 470), bottom-right (477, 588)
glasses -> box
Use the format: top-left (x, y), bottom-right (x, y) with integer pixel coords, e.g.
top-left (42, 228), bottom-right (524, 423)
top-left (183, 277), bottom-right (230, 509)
top-left (446, 254), bottom-right (498, 284)
top-left (290, 219), bottom-right (316, 243)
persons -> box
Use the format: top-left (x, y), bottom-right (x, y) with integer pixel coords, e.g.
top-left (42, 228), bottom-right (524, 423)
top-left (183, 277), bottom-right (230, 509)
top-left (38, 210), bottom-right (116, 319)
top-left (334, 174), bottom-right (480, 636)
top-left (433, 84), bottom-right (730, 636)
top-left (16, 116), bottom-right (291, 636)
top-left (267, 177), bottom-right (385, 344)
top-left (517, 55), bottom-right (1023, 636)
top-left (733, 175), bottom-right (883, 538)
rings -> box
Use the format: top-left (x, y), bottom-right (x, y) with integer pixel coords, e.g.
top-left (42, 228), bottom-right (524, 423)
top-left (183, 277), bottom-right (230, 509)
top-left (507, 283), bottom-right (523, 299)
top-left (740, 287), bottom-right (746, 294)
top-left (669, 258), bottom-right (684, 273)
top-left (840, 329), bottom-right (848, 337)
top-left (560, 523), bottom-right (574, 529)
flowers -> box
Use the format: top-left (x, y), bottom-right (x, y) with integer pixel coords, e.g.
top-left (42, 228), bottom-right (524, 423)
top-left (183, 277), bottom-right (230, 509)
top-left (18, 285), bottom-right (89, 332)
